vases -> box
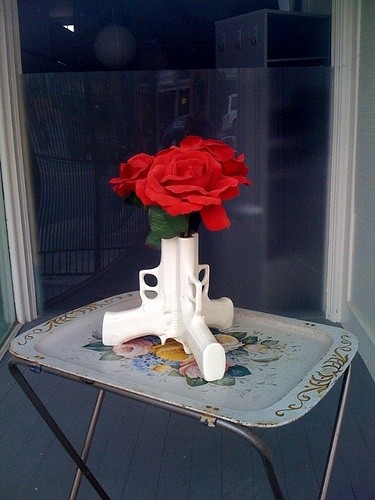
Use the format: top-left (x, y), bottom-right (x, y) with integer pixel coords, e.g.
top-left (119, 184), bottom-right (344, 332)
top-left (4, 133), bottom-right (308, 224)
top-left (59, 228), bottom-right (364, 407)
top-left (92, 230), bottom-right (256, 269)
top-left (98, 231), bottom-right (243, 381)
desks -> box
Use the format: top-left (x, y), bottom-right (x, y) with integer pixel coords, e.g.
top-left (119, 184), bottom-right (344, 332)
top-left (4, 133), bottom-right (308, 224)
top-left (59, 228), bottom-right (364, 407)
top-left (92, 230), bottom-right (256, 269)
top-left (6, 285), bottom-right (357, 499)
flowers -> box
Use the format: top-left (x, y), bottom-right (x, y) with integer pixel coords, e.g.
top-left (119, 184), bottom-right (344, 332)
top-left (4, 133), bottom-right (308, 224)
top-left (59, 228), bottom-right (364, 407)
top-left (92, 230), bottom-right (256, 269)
top-left (111, 137), bottom-right (249, 247)
